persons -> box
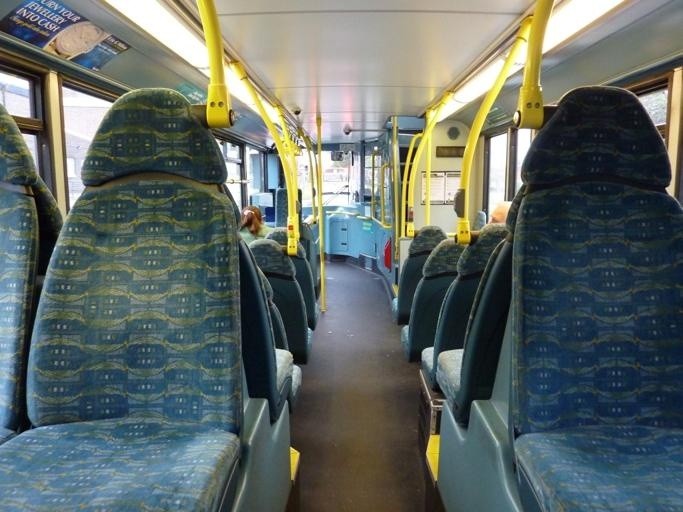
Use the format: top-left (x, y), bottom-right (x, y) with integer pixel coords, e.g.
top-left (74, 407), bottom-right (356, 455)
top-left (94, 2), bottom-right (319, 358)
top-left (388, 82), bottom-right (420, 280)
top-left (240, 206), bottom-right (274, 240)
top-left (490, 200), bottom-right (513, 223)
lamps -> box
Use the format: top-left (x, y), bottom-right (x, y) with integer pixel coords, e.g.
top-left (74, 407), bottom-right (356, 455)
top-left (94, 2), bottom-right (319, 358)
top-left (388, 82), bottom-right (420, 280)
top-left (427, 0), bottom-right (626, 123)
top-left (100, 0), bottom-right (282, 126)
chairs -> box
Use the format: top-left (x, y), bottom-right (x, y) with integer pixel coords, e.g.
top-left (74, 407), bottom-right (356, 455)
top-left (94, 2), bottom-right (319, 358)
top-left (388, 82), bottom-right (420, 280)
top-left (2, 88), bottom-right (321, 512)
top-left (389, 84), bottom-right (681, 510)
